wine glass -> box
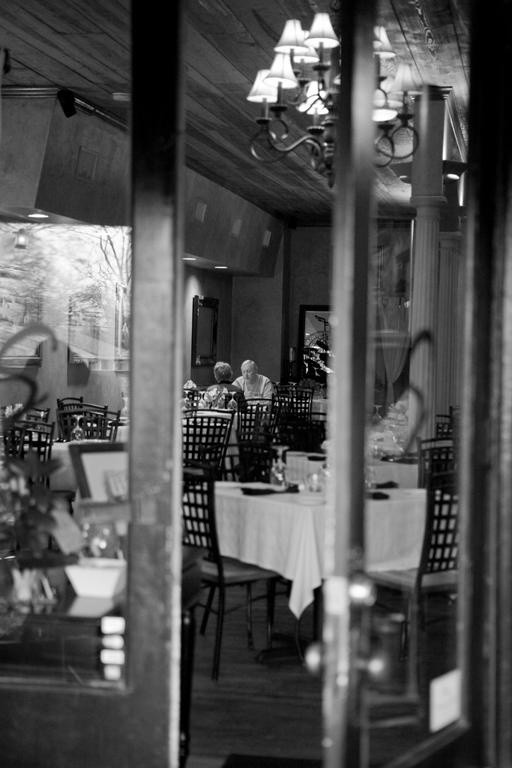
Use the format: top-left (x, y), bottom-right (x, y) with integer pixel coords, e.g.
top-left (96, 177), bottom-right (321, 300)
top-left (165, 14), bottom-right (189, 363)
top-left (370, 405), bottom-right (387, 434)
top-left (70, 415), bottom-right (85, 442)
top-left (120, 396), bottom-right (130, 425)
top-left (287, 380), bottom-right (326, 402)
top-left (196, 388), bottom-right (238, 413)
top-left (266, 440), bottom-right (382, 507)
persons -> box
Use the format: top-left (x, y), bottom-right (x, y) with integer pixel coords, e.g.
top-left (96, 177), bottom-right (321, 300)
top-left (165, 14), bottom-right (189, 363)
top-left (231, 359), bottom-right (275, 404)
top-left (197, 361), bottom-right (249, 412)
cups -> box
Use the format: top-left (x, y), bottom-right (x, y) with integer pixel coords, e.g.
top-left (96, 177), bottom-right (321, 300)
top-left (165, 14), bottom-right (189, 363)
top-left (63, 556), bottom-right (130, 599)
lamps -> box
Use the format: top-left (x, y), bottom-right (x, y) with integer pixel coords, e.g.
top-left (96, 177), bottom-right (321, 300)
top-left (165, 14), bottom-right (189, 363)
top-left (246, 13), bottom-right (419, 187)
